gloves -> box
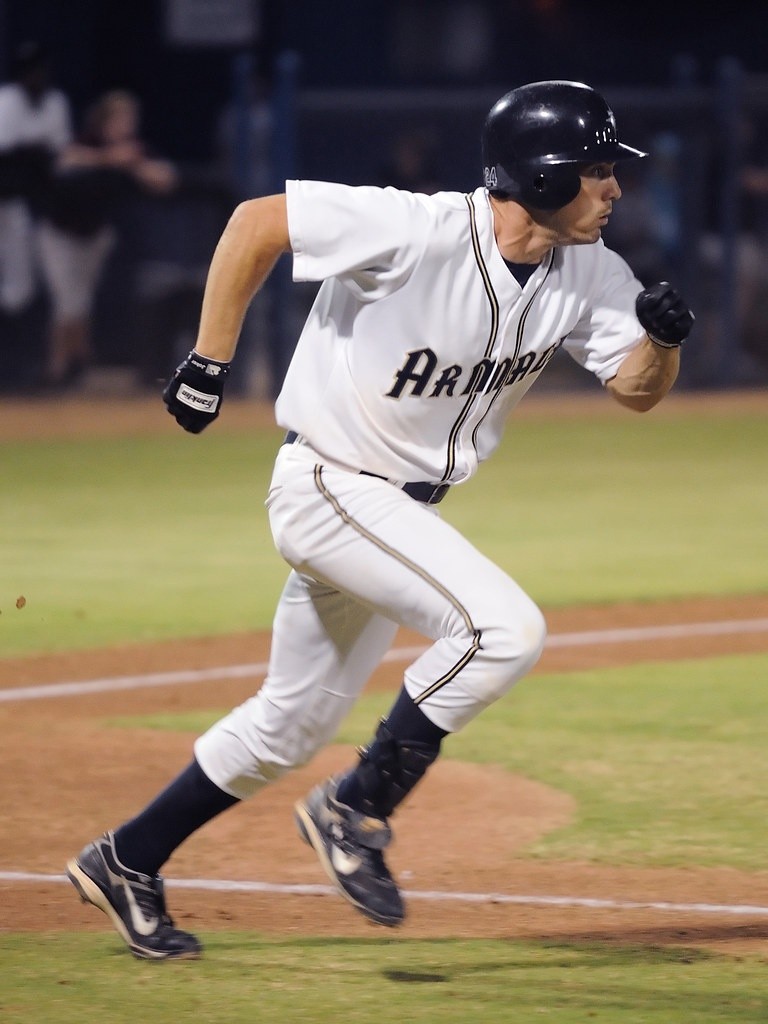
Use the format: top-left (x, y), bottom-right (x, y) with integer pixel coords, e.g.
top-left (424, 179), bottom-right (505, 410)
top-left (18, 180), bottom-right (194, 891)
top-left (163, 348), bottom-right (231, 434)
top-left (636, 281), bottom-right (696, 348)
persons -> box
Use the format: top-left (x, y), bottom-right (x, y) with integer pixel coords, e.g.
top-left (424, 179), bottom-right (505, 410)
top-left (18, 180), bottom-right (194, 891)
top-left (62, 78), bottom-right (698, 960)
top-left (1, 41), bottom-right (178, 397)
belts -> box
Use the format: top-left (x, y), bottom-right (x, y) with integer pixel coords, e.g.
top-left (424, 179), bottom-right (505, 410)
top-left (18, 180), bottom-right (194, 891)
top-left (282, 430), bottom-right (450, 504)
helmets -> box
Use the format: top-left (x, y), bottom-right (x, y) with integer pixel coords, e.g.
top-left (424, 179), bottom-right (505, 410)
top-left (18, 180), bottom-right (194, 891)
top-left (482, 81), bottom-right (649, 209)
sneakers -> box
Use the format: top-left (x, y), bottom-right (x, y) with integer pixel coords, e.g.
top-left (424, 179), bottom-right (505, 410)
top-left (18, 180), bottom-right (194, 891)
top-left (63, 829), bottom-right (201, 961)
top-left (293, 771), bottom-right (405, 929)
